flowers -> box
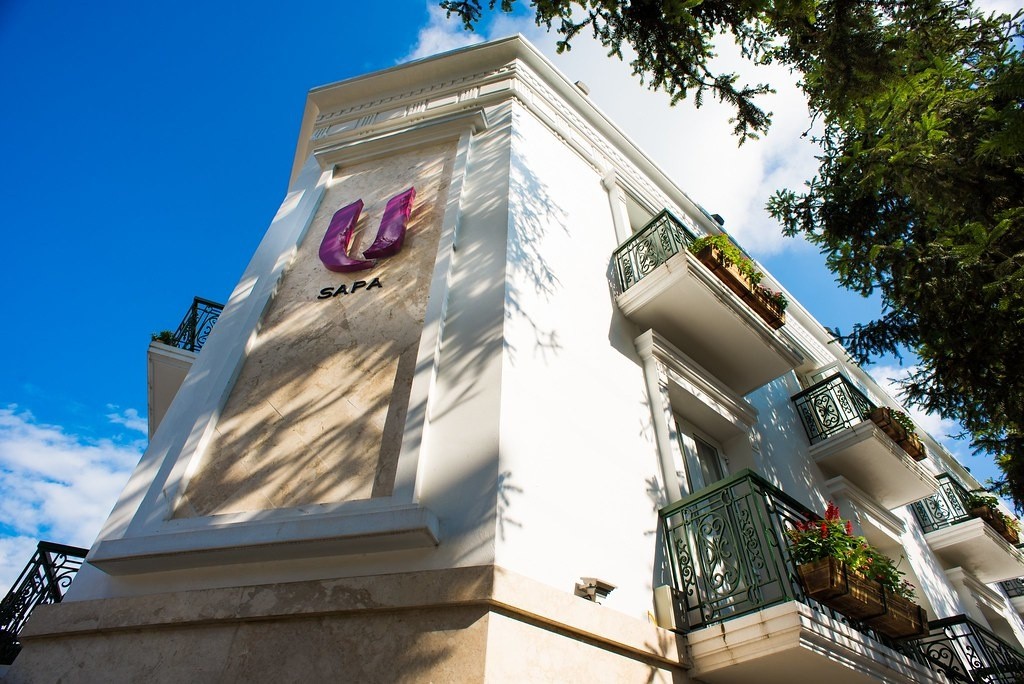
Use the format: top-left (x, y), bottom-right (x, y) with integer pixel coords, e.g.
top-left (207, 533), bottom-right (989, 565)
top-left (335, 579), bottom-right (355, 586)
top-left (690, 231), bottom-right (788, 311)
top-left (862, 405), bottom-right (924, 441)
top-left (782, 500), bottom-right (918, 605)
top-left (969, 495), bottom-right (1021, 532)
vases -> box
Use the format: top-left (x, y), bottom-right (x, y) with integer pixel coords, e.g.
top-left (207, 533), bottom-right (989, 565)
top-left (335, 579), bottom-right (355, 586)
top-left (693, 244), bottom-right (786, 331)
top-left (972, 506), bottom-right (1021, 545)
top-left (795, 557), bottom-right (930, 643)
top-left (867, 407), bottom-right (927, 462)
